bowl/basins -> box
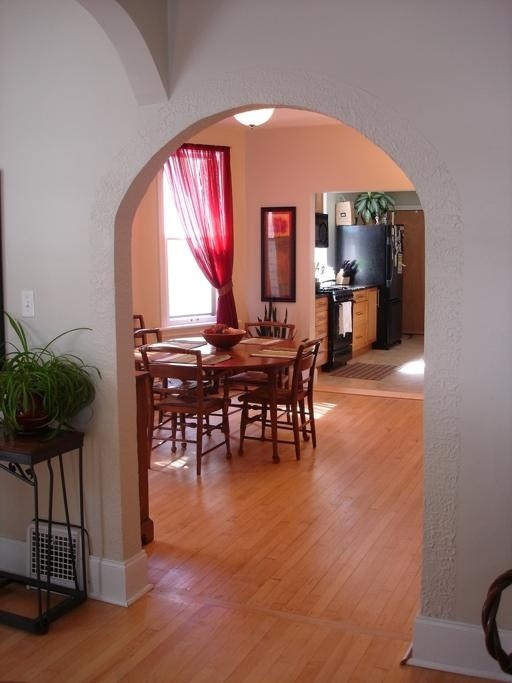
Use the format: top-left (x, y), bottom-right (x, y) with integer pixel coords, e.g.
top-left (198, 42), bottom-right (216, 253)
top-left (200, 328), bottom-right (247, 351)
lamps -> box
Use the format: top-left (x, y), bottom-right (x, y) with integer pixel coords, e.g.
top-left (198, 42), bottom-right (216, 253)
top-left (232, 106), bottom-right (275, 128)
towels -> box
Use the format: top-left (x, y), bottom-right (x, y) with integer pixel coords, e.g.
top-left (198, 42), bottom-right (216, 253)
top-left (338, 299), bottom-right (353, 338)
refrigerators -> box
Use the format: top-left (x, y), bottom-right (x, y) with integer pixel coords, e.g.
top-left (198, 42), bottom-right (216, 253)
top-left (335, 223), bottom-right (403, 343)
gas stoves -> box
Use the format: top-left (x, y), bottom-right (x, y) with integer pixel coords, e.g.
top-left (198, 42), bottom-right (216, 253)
top-left (318, 286), bottom-right (348, 293)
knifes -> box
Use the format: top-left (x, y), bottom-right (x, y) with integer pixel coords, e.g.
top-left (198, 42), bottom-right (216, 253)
top-left (341, 259), bottom-right (358, 277)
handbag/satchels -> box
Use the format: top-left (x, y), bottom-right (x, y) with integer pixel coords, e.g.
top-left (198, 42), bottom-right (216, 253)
top-left (335, 194), bottom-right (356, 226)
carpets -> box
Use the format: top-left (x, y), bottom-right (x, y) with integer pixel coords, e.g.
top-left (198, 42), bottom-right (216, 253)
top-left (330, 362), bottom-right (399, 380)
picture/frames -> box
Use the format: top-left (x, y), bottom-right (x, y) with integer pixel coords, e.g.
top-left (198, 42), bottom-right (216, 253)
top-left (260, 204), bottom-right (299, 300)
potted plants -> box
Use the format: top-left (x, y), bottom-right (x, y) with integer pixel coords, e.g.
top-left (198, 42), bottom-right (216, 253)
top-left (0, 309), bottom-right (100, 438)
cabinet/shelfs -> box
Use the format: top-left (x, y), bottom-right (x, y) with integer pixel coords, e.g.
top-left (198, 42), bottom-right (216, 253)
top-left (135, 371), bottom-right (159, 545)
top-left (315, 193), bottom-right (324, 214)
top-left (314, 295), bottom-right (330, 367)
top-left (0, 431), bottom-right (92, 635)
top-left (354, 285), bottom-right (379, 357)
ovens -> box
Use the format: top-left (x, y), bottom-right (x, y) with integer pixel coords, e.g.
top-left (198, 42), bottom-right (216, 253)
top-left (331, 299), bottom-right (353, 363)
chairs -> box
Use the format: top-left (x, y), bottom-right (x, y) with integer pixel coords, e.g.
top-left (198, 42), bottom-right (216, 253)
top-left (132, 314), bottom-right (321, 475)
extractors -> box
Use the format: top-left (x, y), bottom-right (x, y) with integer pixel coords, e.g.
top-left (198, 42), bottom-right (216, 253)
top-left (315, 213), bottom-right (329, 247)
